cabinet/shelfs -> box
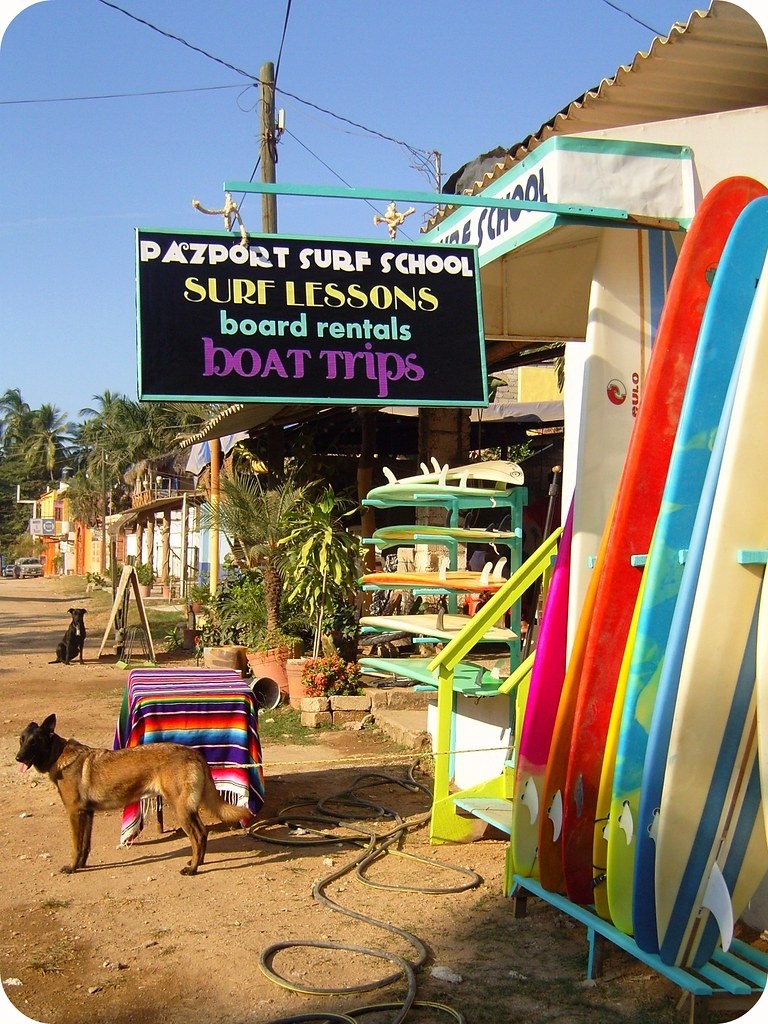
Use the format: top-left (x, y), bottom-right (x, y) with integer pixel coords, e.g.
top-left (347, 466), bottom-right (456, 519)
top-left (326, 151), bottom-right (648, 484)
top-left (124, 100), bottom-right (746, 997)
top-left (355, 457), bottom-right (527, 696)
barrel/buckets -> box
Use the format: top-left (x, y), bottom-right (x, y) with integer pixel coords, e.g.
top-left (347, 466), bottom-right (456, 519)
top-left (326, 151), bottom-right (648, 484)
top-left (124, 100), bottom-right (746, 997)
top-left (243, 676), bottom-right (281, 712)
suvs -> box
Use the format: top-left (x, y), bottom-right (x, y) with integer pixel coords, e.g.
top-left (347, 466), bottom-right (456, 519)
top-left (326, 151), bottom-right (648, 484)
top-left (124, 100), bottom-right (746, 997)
top-left (13, 558), bottom-right (44, 579)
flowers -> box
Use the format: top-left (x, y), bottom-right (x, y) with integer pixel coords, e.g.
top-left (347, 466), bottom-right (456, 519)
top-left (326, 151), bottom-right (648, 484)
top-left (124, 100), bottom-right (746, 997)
top-left (300, 647), bottom-right (361, 697)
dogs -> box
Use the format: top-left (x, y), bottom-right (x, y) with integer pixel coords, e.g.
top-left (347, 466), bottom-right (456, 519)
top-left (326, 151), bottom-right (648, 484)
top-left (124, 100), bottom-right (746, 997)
top-left (48, 608), bottom-right (87, 665)
top-left (17, 714), bottom-right (249, 880)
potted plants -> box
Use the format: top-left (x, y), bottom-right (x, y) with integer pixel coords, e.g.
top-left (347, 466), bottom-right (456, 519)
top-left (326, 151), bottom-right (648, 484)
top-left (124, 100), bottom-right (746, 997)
top-left (131, 559), bottom-right (154, 597)
top-left (163, 573), bottom-right (177, 599)
top-left (279, 483), bottom-right (369, 709)
top-left (183, 461), bottom-right (299, 698)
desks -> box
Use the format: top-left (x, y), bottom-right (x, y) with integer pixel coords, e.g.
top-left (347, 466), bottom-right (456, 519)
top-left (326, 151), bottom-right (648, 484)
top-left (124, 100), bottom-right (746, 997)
top-left (112, 668), bottom-right (265, 851)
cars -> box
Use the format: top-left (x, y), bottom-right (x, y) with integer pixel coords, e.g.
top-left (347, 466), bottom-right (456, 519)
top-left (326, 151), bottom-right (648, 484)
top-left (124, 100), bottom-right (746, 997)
top-left (5, 565), bottom-right (16, 576)
